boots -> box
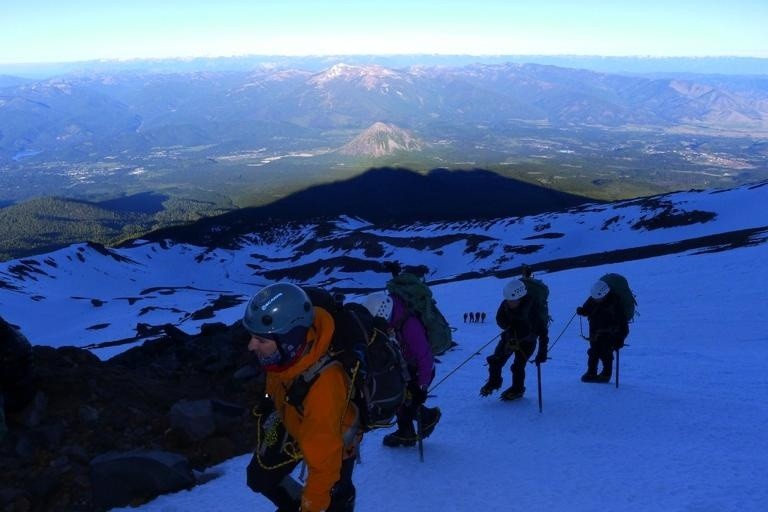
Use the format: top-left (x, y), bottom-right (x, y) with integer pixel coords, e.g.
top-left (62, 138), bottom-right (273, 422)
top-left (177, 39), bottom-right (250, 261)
top-left (501, 364), bottom-right (526, 400)
top-left (582, 348), bottom-right (597, 382)
top-left (593, 349), bottom-right (613, 382)
top-left (478, 355), bottom-right (502, 395)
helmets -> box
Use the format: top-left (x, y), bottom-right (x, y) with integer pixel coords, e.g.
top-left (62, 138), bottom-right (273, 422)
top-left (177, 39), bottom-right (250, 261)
top-left (244, 282), bottom-right (314, 341)
top-left (363, 293), bottom-right (392, 322)
top-left (590, 281), bottom-right (608, 298)
top-left (502, 280), bottom-right (526, 302)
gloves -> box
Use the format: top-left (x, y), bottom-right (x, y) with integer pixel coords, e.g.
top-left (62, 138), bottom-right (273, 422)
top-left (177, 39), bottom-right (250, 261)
top-left (535, 354), bottom-right (546, 364)
top-left (577, 307), bottom-right (584, 315)
top-left (613, 340), bottom-right (623, 350)
top-left (412, 391), bottom-right (427, 403)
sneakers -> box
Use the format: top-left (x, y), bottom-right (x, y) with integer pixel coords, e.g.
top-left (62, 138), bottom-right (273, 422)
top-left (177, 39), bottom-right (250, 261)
top-left (419, 408), bottom-right (441, 440)
top-left (384, 432), bottom-right (417, 447)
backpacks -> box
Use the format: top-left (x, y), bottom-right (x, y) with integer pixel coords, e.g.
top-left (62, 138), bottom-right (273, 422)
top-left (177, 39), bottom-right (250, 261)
top-left (601, 273), bottom-right (638, 324)
top-left (285, 294), bottom-right (406, 432)
top-left (519, 275), bottom-right (549, 310)
top-left (387, 273), bottom-right (454, 352)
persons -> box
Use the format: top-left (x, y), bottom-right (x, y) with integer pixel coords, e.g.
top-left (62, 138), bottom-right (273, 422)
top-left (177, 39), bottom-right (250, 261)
top-left (359, 291), bottom-right (441, 449)
top-left (243, 283), bottom-right (363, 512)
top-left (479, 279), bottom-right (549, 400)
top-left (463, 311), bottom-right (487, 323)
top-left (576, 280), bottom-right (629, 384)
